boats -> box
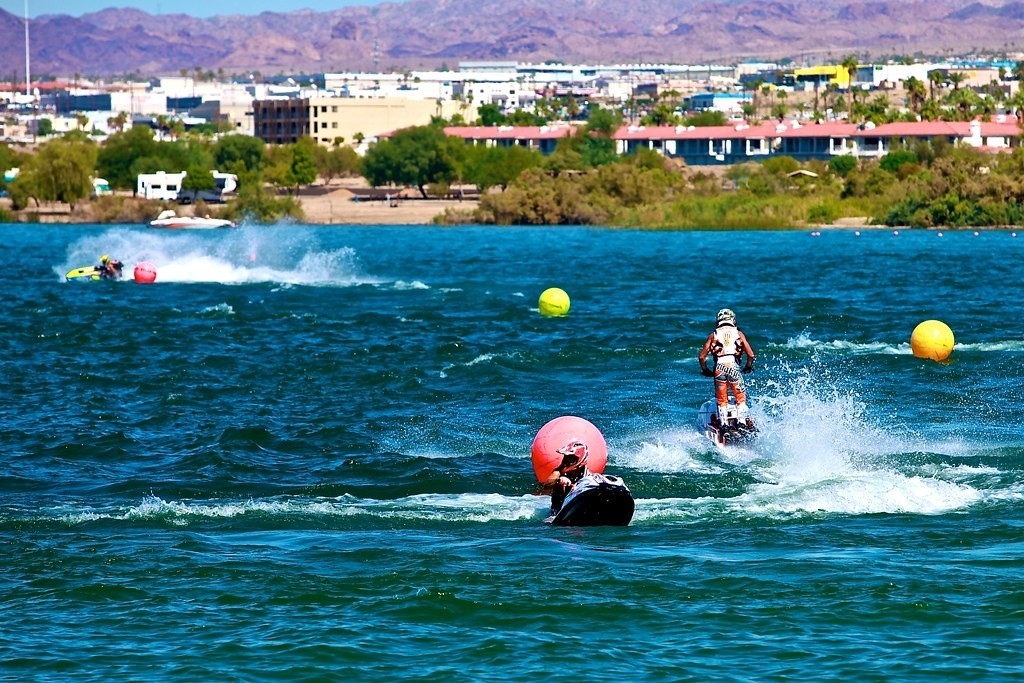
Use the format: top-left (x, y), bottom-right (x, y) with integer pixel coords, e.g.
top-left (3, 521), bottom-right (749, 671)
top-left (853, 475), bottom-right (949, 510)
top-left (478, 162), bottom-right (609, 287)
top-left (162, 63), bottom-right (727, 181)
top-left (149, 210), bottom-right (231, 230)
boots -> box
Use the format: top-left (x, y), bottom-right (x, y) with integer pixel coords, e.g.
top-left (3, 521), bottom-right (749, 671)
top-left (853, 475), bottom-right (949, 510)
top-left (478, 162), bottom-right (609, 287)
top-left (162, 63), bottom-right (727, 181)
top-left (736, 402), bottom-right (748, 427)
top-left (718, 406), bottom-right (729, 427)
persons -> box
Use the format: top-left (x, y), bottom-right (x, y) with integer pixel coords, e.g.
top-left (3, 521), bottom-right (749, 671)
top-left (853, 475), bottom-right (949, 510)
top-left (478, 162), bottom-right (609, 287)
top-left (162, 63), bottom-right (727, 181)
top-left (543, 441), bottom-right (592, 523)
top-left (699, 308), bottom-right (754, 428)
top-left (99, 256), bottom-right (117, 274)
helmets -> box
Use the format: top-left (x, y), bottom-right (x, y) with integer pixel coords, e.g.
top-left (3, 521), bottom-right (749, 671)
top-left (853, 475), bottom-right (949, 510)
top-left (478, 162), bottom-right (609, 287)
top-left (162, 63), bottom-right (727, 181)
top-left (99, 255), bottom-right (110, 265)
top-left (716, 308), bottom-right (736, 327)
top-left (556, 438), bottom-right (588, 475)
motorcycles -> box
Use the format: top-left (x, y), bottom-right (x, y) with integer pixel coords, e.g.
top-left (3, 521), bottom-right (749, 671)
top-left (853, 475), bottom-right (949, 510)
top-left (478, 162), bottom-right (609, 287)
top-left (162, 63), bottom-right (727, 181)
top-left (699, 367), bottom-right (762, 448)
top-left (550, 472), bottom-right (634, 526)
top-left (65, 259), bottom-right (124, 282)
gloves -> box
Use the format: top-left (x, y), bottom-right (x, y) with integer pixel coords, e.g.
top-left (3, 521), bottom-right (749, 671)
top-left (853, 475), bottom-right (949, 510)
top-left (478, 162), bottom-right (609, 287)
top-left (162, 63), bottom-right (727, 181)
top-left (559, 476), bottom-right (571, 487)
top-left (742, 365), bottom-right (752, 374)
top-left (703, 368), bottom-right (712, 377)
